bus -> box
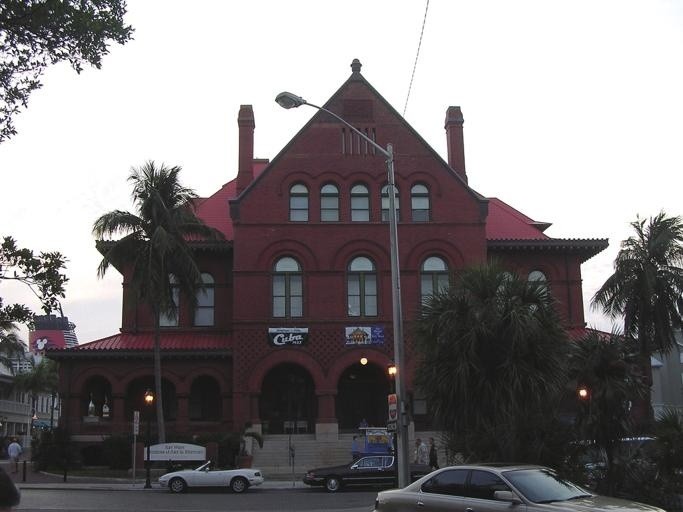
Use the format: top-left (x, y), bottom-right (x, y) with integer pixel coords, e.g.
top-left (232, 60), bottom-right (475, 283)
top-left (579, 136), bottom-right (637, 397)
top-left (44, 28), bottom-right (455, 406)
top-left (564, 436), bottom-right (661, 483)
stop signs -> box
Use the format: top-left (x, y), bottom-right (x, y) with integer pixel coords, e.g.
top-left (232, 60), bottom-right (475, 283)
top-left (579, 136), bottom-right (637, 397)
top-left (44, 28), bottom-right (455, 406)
top-left (388, 395), bottom-right (396, 419)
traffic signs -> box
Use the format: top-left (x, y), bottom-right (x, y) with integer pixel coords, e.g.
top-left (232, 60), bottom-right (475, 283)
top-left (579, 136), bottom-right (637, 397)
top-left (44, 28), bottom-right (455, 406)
top-left (386, 421), bottom-right (398, 434)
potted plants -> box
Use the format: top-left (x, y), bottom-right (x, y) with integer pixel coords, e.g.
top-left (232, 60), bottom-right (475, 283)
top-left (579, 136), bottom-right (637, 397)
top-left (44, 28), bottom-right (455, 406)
top-left (224, 421), bottom-right (264, 468)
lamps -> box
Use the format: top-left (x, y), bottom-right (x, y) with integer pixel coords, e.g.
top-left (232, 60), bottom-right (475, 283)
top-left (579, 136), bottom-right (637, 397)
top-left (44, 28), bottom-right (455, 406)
top-left (359, 354), bottom-right (369, 366)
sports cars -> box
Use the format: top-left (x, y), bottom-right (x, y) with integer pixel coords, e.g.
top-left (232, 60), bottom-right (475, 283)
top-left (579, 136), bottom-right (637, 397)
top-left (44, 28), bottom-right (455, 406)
top-left (158, 461), bottom-right (264, 493)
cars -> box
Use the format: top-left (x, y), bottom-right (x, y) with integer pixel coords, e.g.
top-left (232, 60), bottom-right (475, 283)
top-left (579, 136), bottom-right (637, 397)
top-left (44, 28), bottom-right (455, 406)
top-left (303, 453), bottom-right (430, 492)
top-left (371, 465), bottom-right (669, 512)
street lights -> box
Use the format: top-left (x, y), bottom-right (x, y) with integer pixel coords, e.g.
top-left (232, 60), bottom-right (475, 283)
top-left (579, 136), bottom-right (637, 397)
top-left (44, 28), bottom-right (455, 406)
top-left (144, 389), bottom-right (155, 488)
top-left (274, 91), bottom-right (415, 491)
top-left (579, 384), bottom-right (593, 416)
top-left (388, 356), bottom-right (397, 453)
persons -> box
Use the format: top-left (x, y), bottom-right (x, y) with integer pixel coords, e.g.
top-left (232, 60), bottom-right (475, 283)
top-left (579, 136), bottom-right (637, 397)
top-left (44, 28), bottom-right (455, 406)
top-left (355, 417), bottom-right (369, 433)
top-left (0, 464), bottom-right (20, 511)
top-left (349, 435), bottom-right (361, 459)
top-left (6, 436), bottom-right (23, 474)
top-left (413, 438), bottom-right (430, 465)
top-left (428, 437), bottom-right (439, 471)
